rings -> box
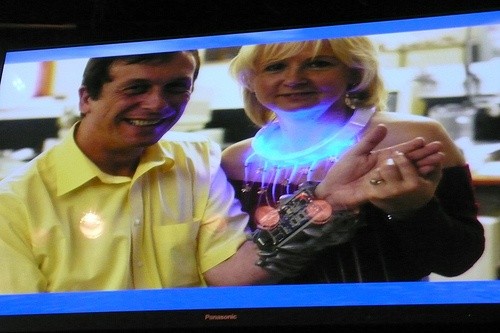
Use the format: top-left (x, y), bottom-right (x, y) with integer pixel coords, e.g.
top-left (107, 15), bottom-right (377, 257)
top-left (369, 177), bottom-right (382, 184)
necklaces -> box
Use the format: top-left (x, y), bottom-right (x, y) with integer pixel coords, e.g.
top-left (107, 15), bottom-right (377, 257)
top-left (242, 105), bottom-right (380, 210)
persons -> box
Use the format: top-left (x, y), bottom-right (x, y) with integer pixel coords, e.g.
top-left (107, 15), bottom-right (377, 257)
top-left (218, 35), bottom-right (486, 289)
top-left (1, 49), bottom-right (446, 296)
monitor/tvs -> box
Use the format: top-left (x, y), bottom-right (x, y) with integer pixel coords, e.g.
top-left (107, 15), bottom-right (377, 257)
top-left (0, 7), bottom-right (500, 331)
